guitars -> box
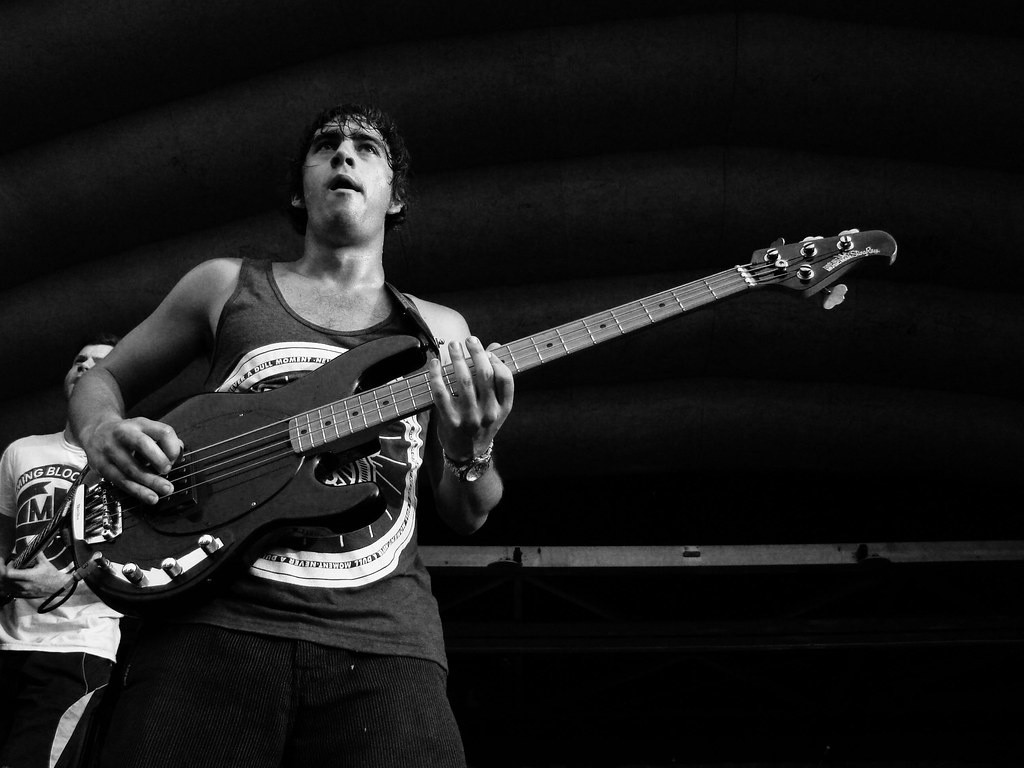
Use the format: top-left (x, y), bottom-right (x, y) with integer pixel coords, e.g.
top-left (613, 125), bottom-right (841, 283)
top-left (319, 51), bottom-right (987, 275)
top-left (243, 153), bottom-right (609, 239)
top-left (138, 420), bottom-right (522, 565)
top-left (0, 495), bottom-right (67, 608)
top-left (67, 223), bottom-right (900, 620)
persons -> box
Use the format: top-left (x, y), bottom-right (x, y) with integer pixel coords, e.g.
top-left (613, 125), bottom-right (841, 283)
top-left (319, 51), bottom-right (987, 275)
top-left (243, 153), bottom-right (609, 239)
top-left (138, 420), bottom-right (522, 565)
top-left (0, 332), bottom-right (122, 768)
top-left (69, 104), bottom-right (514, 768)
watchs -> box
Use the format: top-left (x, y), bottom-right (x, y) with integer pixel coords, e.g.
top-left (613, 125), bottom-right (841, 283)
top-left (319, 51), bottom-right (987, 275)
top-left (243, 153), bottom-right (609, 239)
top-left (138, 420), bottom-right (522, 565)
top-left (442, 441), bottom-right (494, 482)
